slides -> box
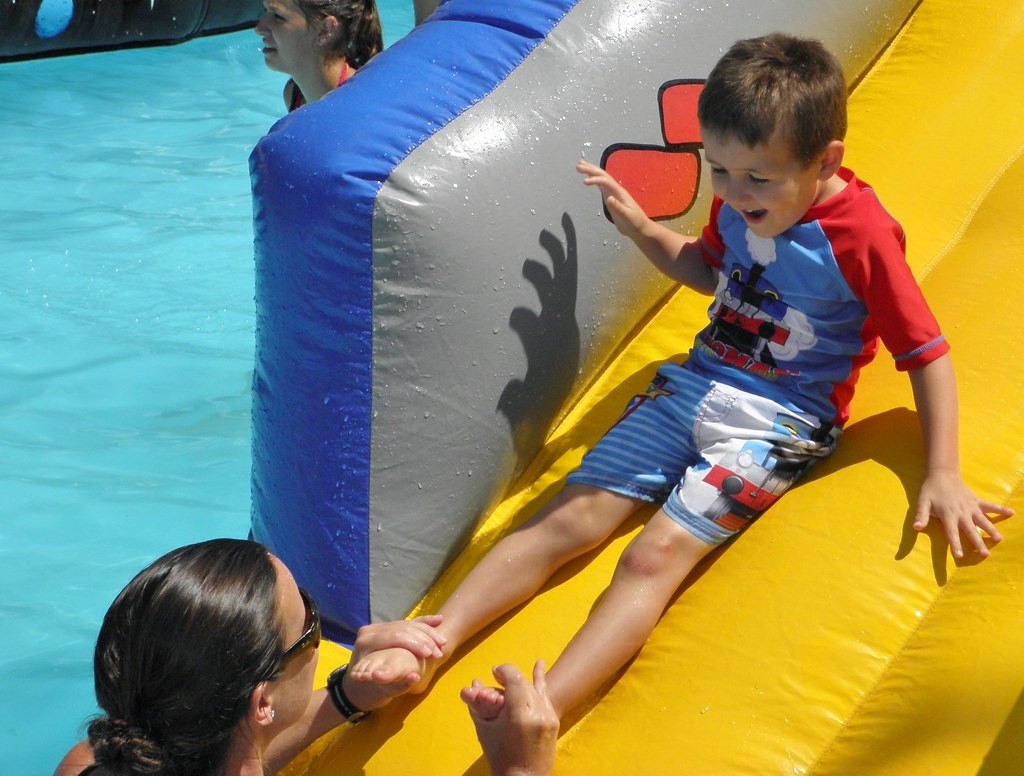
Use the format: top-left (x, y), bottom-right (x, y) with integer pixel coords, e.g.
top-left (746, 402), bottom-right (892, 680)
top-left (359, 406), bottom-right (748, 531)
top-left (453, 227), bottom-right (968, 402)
top-left (248, 0), bottom-right (1024, 774)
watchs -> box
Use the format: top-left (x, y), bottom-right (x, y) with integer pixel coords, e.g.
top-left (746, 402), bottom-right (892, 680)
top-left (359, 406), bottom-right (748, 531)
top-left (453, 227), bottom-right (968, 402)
top-left (326, 664), bottom-right (372, 727)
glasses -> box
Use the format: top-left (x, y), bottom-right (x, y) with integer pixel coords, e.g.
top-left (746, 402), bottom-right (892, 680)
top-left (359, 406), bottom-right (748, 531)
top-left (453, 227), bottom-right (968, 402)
top-left (284, 588), bottom-right (321, 656)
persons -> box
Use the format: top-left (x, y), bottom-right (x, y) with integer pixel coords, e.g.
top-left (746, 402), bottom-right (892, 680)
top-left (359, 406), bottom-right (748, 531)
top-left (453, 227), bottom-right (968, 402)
top-left (348, 34), bottom-right (1013, 734)
top-left (56, 539), bottom-right (561, 775)
top-left (253, 0), bottom-right (444, 116)
